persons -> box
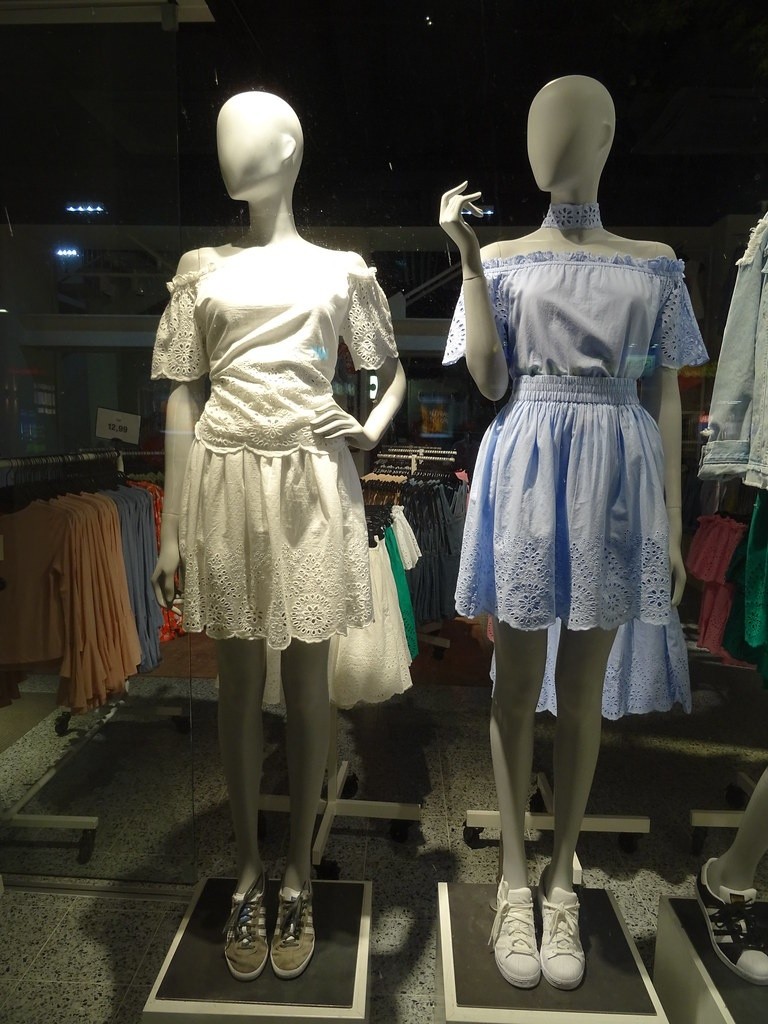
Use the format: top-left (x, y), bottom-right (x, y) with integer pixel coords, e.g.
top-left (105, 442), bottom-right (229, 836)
top-left (694, 767), bottom-right (768, 986)
top-left (153, 91), bottom-right (407, 982)
top-left (440, 75), bottom-right (708, 990)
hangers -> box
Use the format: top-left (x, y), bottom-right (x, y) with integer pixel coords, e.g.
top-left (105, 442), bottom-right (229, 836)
top-left (362, 445), bottom-right (463, 539)
top-left (0, 448), bottom-right (166, 513)
top-left (714, 507), bottom-right (753, 524)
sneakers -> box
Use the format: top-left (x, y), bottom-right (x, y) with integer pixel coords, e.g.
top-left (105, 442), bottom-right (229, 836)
top-left (537, 864), bottom-right (585, 990)
top-left (488, 875), bottom-right (541, 988)
top-left (222, 863), bottom-right (269, 981)
top-left (695, 857), bottom-right (768, 984)
top-left (270, 872), bottom-right (315, 978)
